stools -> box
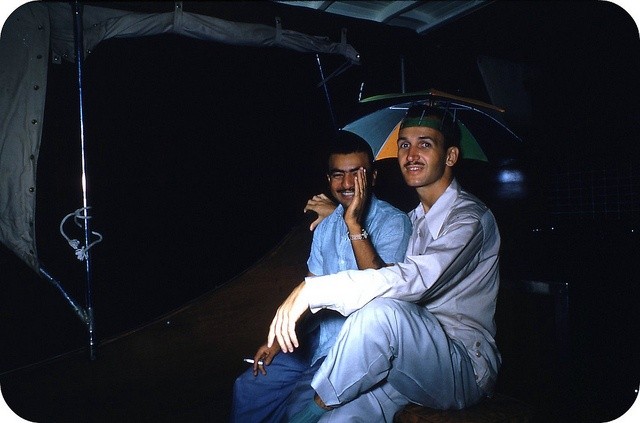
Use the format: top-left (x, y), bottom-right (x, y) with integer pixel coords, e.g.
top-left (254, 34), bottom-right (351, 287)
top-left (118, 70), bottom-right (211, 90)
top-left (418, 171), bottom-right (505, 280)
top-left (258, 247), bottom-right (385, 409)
top-left (393, 394), bottom-right (537, 423)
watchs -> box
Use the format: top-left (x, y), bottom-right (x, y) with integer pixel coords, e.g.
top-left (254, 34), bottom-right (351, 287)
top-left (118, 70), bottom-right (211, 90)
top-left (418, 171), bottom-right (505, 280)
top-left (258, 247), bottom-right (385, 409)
top-left (349, 228), bottom-right (370, 240)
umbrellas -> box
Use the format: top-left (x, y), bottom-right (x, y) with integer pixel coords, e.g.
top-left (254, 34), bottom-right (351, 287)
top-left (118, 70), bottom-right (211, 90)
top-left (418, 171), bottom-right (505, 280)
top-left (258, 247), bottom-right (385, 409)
top-left (337, 97), bottom-right (499, 194)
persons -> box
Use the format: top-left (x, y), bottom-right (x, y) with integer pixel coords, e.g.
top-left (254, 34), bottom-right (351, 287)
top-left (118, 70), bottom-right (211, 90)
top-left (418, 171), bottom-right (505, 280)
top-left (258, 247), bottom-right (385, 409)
top-left (267, 104), bottom-right (504, 422)
top-left (229, 137), bottom-right (411, 422)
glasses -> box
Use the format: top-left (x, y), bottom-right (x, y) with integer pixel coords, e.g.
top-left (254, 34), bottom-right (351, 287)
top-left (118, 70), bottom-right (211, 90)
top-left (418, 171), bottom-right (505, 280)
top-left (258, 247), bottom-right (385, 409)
top-left (328, 171), bottom-right (357, 180)
